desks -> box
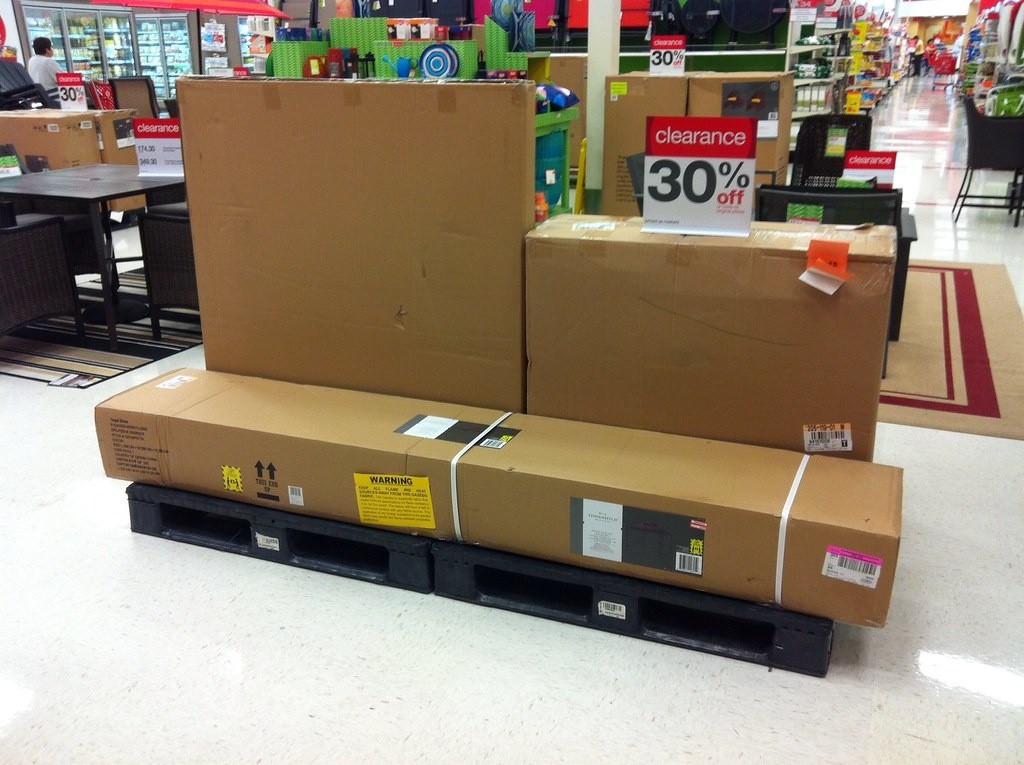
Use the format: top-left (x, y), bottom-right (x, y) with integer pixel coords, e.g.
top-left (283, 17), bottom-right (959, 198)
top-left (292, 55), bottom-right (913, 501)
top-left (0, 163), bottom-right (184, 351)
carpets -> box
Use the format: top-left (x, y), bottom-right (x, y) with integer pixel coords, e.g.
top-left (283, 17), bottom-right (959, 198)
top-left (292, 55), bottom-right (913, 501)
top-left (878, 260), bottom-right (1024, 441)
top-left (0, 266), bottom-right (203, 389)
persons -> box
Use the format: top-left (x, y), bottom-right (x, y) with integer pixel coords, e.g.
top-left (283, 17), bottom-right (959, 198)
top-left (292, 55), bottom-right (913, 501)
top-left (911, 35), bottom-right (925, 76)
top-left (27, 37), bottom-right (66, 110)
top-left (923, 39), bottom-right (939, 74)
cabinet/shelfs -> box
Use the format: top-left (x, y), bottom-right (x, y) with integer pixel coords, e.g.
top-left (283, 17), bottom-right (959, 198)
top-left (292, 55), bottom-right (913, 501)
top-left (783, 6), bottom-right (909, 162)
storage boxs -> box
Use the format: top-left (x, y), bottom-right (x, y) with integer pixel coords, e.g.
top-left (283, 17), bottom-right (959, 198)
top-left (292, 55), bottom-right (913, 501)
top-left (0, 107), bottom-right (145, 212)
top-left (96, 8), bottom-right (903, 627)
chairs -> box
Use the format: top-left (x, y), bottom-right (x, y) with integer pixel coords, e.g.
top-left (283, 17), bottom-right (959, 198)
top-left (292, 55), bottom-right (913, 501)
top-left (951, 95), bottom-right (1024, 228)
top-left (137, 213), bottom-right (201, 339)
top-left (0, 61), bottom-right (58, 110)
top-left (109, 76), bottom-right (161, 119)
top-left (0, 201), bottom-right (86, 343)
